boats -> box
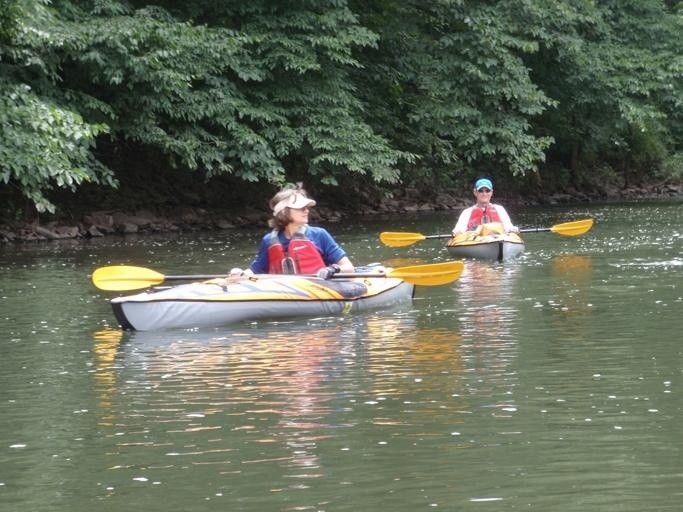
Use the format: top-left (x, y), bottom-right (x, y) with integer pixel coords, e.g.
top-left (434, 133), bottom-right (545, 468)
top-left (109, 261), bottom-right (424, 333)
top-left (441, 227), bottom-right (528, 262)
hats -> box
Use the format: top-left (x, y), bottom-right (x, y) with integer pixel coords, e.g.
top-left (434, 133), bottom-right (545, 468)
top-left (272, 191), bottom-right (316, 217)
top-left (475, 179), bottom-right (493, 192)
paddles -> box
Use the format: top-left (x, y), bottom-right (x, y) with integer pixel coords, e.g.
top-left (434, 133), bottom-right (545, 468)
top-left (380, 218), bottom-right (594, 249)
top-left (92, 261), bottom-right (464, 292)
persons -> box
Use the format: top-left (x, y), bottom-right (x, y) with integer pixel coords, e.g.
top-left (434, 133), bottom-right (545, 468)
top-left (451, 179), bottom-right (519, 234)
top-left (226, 182), bottom-right (355, 280)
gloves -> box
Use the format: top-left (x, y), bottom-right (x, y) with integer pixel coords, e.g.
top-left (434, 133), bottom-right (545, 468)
top-left (317, 264), bottom-right (340, 281)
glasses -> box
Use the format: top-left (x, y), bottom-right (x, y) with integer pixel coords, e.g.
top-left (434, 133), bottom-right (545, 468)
top-left (477, 188), bottom-right (490, 194)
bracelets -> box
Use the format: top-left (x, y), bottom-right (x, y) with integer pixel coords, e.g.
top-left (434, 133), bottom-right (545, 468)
top-left (331, 263), bottom-right (340, 273)
